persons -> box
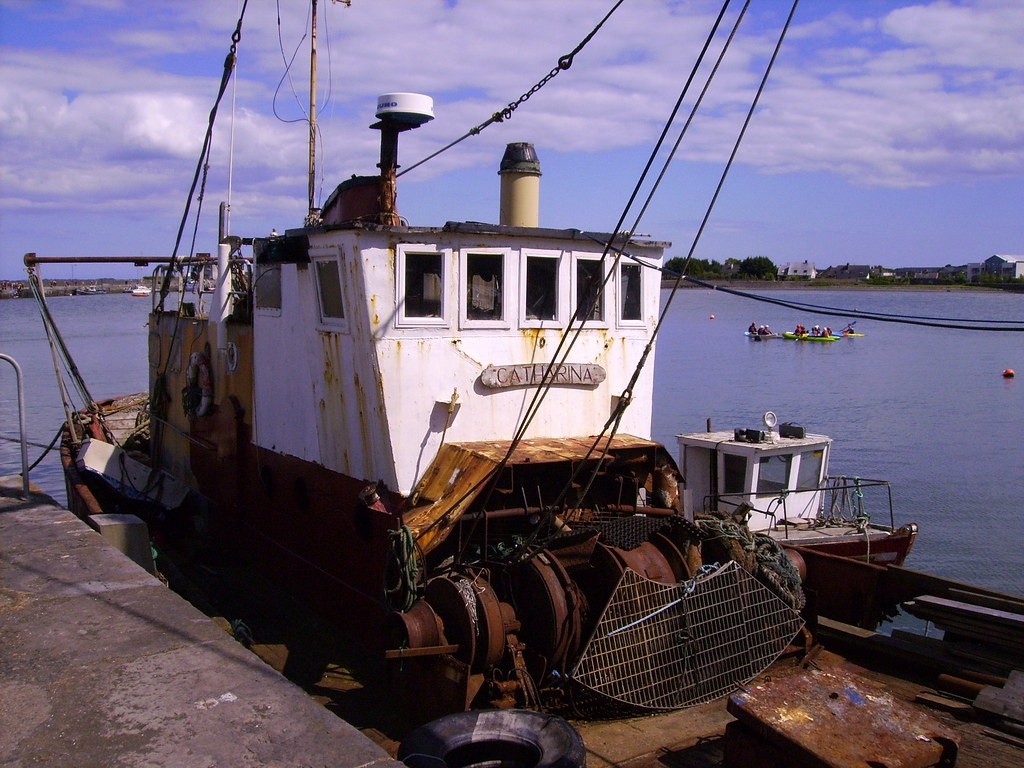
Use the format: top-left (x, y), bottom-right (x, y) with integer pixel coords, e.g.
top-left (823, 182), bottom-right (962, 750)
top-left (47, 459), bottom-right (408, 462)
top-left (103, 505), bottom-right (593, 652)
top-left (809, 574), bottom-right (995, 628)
top-left (794, 324), bottom-right (833, 338)
top-left (748, 321), bottom-right (758, 334)
top-left (764, 324), bottom-right (773, 335)
top-left (842, 323), bottom-right (855, 334)
top-left (758, 325), bottom-right (764, 334)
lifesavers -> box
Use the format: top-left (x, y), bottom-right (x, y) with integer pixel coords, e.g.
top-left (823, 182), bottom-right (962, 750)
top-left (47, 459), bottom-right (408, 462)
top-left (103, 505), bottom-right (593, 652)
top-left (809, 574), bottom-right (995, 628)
top-left (397, 708), bottom-right (585, 768)
top-left (186, 352), bottom-right (212, 416)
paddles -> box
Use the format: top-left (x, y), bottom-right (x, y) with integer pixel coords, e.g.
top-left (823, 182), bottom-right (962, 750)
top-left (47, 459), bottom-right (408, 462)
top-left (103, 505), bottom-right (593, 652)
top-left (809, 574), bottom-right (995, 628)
top-left (837, 321), bottom-right (856, 332)
top-left (795, 334), bottom-right (801, 342)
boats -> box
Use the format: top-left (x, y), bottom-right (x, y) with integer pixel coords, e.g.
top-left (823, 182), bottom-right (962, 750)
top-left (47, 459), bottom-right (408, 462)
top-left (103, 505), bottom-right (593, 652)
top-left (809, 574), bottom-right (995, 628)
top-left (783, 331), bottom-right (865, 341)
top-left (744, 329), bottom-right (779, 338)
top-left (70, 285), bottom-right (105, 295)
top-left (124, 279), bottom-right (215, 297)
top-left (23, 0), bottom-right (1024, 767)
top-left (668, 412), bottom-right (917, 568)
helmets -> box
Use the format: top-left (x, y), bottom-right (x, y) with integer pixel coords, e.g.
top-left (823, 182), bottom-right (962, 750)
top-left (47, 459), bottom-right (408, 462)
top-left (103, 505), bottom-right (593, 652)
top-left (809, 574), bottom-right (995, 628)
top-left (752, 322), bottom-right (756, 325)
top-left (760, 325), bottom-right (764, 329)
top-left (800, 325), bottom-right (804, 327)
top-left (823, 326), bottom-right (827, 331)
top-left (815, 326), bottom-right (818, 330)
top-left (765, 325), bottom-right (769, 327)
top-left (797, 324), bottom-right (800, 328)
top-left (828, 327), bottom-right (831, 330)
